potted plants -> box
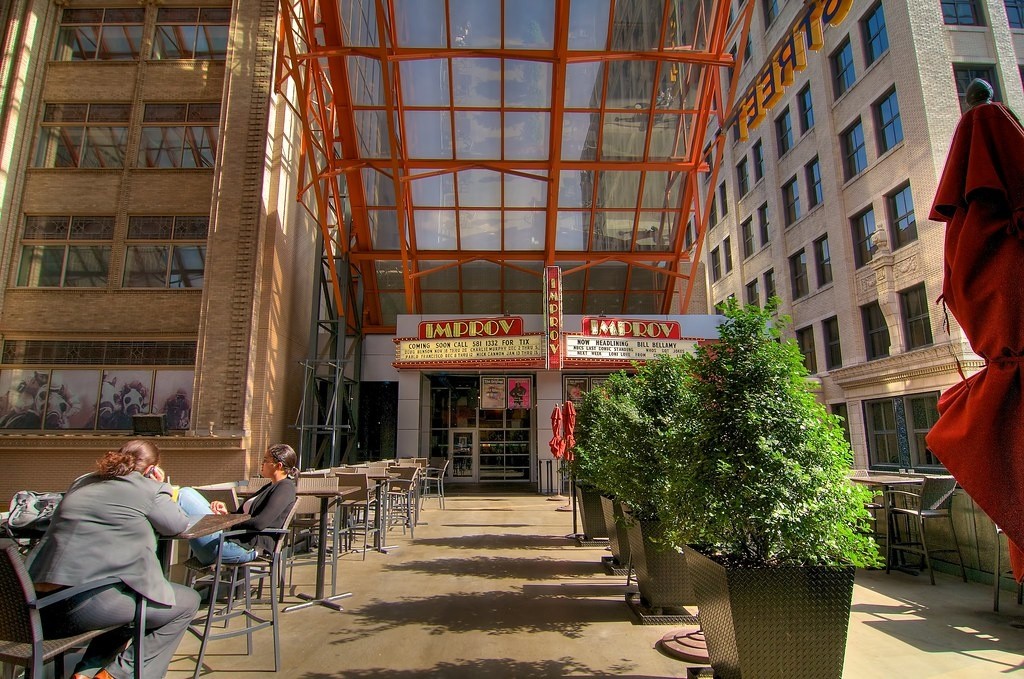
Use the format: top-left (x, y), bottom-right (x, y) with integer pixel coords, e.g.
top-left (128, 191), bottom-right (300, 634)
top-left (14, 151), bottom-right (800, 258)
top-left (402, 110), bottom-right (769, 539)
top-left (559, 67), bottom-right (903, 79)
top-left (559, 388), bottom-right (611, 548)
top-left (588, 375), bottom-right (637, 575)
top-left (614, 352), bottom-right (700, 627)
top-left (663, 295), bottom-right (885, 679)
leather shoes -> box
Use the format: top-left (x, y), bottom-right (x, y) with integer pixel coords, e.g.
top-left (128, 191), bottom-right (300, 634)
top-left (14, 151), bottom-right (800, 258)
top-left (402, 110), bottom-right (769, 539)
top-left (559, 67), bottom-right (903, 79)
top-left (68, 667), bottom-right (114, 679)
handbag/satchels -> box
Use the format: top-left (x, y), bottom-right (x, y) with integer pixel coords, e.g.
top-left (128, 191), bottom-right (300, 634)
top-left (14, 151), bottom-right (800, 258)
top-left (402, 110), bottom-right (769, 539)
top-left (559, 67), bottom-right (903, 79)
top-left (8, 489), bottom-right (66, 530)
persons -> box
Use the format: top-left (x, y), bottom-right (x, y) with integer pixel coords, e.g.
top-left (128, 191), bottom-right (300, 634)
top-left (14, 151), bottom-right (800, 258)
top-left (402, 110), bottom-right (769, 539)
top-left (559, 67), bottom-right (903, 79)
top-left (161, 388), bottom-right (192, 424)
top-left (24, 439), bottom-right (201, 679)
top-left (177, 445), bottom-right (298, 563)
top-left (14, 371), bottom-right (82, 419)
top-left (510, 382), bottom-right (526, 407)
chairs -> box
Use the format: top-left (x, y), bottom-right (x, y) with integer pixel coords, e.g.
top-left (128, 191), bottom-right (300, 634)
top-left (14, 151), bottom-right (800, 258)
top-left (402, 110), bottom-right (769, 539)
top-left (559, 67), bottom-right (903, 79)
top-left (451, 448), bottom-right (471, 476)
top-left (991, 521), bottom-right (1020, 611)
top-left (889, 476), bottom-right (967, 585)
top-left (843, 469), bottom-right (887, 570)
top-left (0, 457), bottom-right (450, 679)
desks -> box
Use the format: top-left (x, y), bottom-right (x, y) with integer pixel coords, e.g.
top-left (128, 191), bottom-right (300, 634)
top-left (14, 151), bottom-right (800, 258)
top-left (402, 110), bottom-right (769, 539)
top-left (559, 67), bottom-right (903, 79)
top-left (367, 466), bottom-right (429, 529)
top-left (846, 477), bottom-right (923, 576)
top-left (234, 485), bottom-right (362, 612)
top-left (0, 513), bottom-right (251, 583)
top-left (326, 473), bottom-right (402, 555)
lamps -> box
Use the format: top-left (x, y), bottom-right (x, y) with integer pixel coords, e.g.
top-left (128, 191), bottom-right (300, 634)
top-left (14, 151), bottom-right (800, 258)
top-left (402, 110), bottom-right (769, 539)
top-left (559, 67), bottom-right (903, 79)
top-left (132, 413), bottom-right (168, 436)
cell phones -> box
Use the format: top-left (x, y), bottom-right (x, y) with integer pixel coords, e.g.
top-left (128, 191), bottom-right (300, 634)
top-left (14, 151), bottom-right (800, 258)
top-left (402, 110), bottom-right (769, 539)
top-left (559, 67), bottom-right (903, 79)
top-left (145, 468), bottom-right (154, 478)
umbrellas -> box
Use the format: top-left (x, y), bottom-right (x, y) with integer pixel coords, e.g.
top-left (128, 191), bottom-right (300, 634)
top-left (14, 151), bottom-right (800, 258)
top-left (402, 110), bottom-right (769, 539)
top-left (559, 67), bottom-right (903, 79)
top-left (546, 404), bottom-right (565, 501)
top-left (563, 401), bottom-right (585, 539)
top-left (923, 78), bottom-right (1024, 590)
